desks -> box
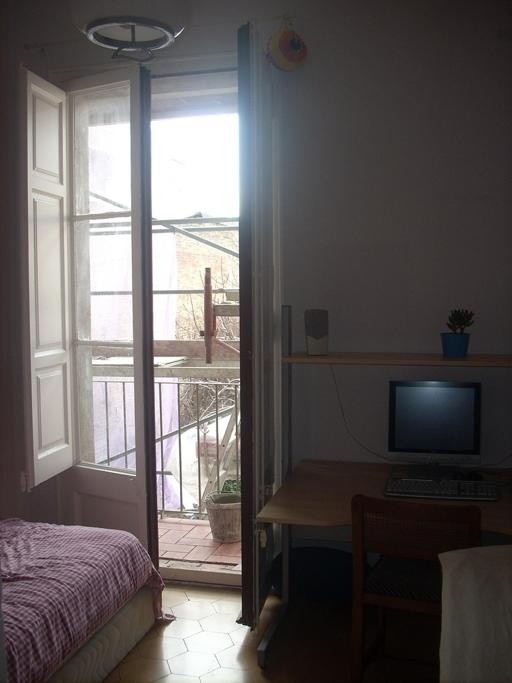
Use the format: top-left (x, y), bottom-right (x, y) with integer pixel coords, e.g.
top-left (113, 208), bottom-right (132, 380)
top-left (257, 459), bottom-right (512, 670)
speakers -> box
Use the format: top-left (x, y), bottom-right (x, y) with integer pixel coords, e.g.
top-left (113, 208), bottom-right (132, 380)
top-left (304, 309), bottom-right (328, 356)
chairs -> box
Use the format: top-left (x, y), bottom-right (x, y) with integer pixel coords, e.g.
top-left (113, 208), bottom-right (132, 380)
top-left (351, 494), bottom-right (482, 682)
top-left (437, 545), bottom-right (512, 682)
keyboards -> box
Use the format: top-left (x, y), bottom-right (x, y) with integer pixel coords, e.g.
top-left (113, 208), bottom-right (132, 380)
top-left (383, 478), bottom-right (500, 502)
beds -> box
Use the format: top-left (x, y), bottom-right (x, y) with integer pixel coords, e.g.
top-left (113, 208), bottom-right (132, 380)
top-left (0, 519), bottom-right (174, 682)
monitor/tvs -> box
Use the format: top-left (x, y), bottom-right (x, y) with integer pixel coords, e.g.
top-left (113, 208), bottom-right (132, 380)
top-left (384, 376), bottom-right (485, 479)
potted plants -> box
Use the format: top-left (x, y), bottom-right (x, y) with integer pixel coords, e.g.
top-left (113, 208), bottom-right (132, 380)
top-left (439, 308), bottom-right (475, 356)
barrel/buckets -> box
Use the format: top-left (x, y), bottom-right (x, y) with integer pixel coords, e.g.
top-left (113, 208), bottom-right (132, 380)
top-left (204, 490), bottom-right (242, 544)
